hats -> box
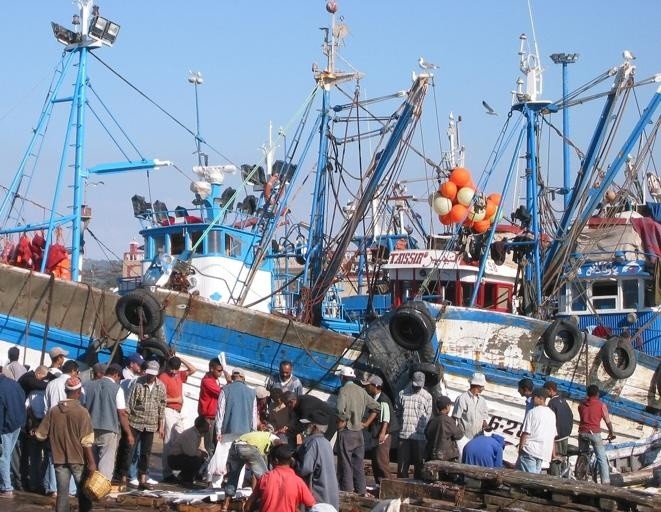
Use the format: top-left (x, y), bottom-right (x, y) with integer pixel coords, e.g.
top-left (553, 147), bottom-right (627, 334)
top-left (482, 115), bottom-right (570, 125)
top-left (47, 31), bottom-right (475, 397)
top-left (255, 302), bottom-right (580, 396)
top-left (468, 373), bottom-right (488, 387)
top-left (309, 503), bottom-right (337, 512)
top-left (299, 410), bottom-right (330, 425)
top-left (92, 352), bottom-right (160, 379)
top-left (65, 377), bottom-right (82, 391)
top-left (255, 386), bottom-right (271, 399)
top-left (340, 367), bottom-right (356, 379)
top-left (49, 347), bottom-right (69, 359)
top-left (412, 371), bottom-right (425, 387)
top-left (528, 388), bottom-right (548, 398)
top-left (360, 376), bottom-right (383, 387)
top-left (232, 368), bottom-right (245, 381)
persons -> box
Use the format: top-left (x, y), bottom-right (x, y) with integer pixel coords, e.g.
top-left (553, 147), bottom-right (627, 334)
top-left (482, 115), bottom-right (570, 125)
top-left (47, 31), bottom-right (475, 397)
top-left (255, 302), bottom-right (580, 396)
top-left (2, 347), bottom-right (340, 511)
top-left (514, 378), bottom-right (614, 486)
top-left (333, 364), bottom-right (505, 498)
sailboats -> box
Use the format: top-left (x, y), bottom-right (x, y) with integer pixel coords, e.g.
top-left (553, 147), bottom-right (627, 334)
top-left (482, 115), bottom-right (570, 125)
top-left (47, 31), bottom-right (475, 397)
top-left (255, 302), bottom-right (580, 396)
top-left (362, 28), bottom-right (661, 490)
top-left (0, 1), bottom-right (659, 493)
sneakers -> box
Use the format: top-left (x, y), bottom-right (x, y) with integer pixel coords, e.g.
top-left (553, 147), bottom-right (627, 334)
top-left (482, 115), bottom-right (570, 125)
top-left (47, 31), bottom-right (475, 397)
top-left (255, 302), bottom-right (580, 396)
top-left (138, 483), bottom-right (154, 490)
top-left (129, 478), bottom-right (139, 486)
top-left (146, 478), bottom-right (159, 485)
top-left (119, 481), bottom-right (128, 492)
top-left (0, 491), bottom-right (14, 499)
top-left (52, 492), bottom-right (57, 498)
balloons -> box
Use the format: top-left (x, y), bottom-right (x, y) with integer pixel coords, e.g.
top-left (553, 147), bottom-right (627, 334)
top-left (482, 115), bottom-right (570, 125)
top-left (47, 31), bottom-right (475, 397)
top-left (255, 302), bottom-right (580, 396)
top-left (428, 168), bottom-right (502, 233)
